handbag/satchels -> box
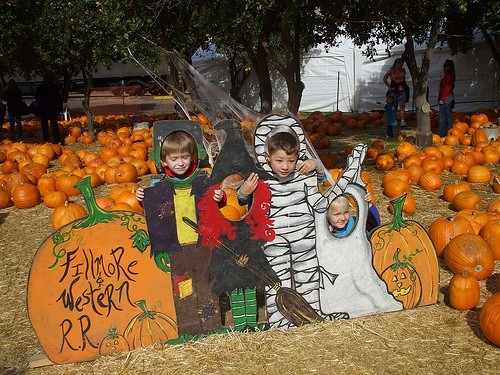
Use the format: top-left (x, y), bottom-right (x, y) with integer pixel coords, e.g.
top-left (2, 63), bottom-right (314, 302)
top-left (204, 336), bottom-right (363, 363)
top-left (21, 102), bottom-right (29, 115)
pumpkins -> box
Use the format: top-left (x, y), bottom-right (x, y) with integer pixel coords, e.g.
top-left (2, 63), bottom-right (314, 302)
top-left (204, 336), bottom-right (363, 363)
top-left (299, 110), bottom-right (500, 280)
top-left (0, 110), bottom-right (260, 231)
top-left (448, 268), bottom-right (480, 310)
top-left (480, 292), bottom-right (500, 347)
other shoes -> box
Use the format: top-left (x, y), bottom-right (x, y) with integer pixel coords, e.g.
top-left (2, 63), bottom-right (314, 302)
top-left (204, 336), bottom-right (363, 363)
top-left (29, 101), bottom-right (39, 116)
top-left (394, 121), bottom-right (398, 126)
top-left (400, 121), bottom-right (407, 127)
top-left (386, 135), bottom-right (395, 139)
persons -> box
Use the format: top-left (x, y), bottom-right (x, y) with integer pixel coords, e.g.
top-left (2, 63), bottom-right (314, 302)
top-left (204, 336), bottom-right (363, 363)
top-left (238, 131), bottom-right (325, 205)
top-left (437, 59), bottom-right (456, 137)
top-left (134, 131), bottom-right (227, 208)
top-left (327, 190), bottom-right (381, 238)
top-left (376, 96), bottom-right (396, 138)
top-left (382, 58), bottom-right (407, 126)
top-left (1, 78), bottom-right (24, 142)
top-left (35, 72), bottom-right (63, 145)
